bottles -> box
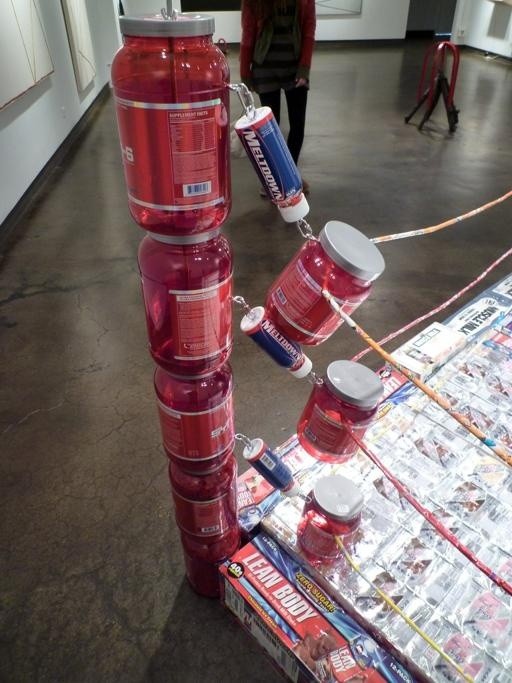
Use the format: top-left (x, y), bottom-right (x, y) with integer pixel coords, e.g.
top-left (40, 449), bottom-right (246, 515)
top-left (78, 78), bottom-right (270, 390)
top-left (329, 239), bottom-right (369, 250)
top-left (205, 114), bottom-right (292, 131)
top-left (139, 233), bottom-right (235, 376)
top-left (264, 220), bottom-right (386, 348)
top-left (298, 361), bottom-right (386, 465)
top-left (167, 466), bottom-right (240, 539)
top-left (297, 475), bottom-right (358, 568)
top-left (181, 521), bottom-right (242, 597)
top-left (111, 13), bottom-right (232, 234)
top-left (154, 363), bottom-right (234, 478)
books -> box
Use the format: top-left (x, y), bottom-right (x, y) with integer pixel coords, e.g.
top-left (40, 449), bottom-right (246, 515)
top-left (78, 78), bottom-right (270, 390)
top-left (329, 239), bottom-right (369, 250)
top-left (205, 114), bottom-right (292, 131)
top-left (386, 321), bottom-right (466, 380)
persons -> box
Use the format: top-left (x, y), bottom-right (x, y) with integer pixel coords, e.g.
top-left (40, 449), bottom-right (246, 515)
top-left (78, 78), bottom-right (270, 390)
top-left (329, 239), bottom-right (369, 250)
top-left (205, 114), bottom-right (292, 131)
top-left (240, 0), bottom-right (316, 199)
